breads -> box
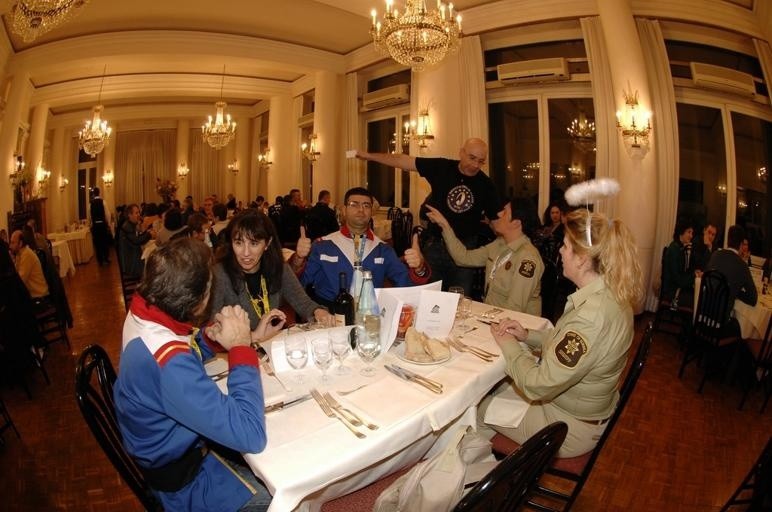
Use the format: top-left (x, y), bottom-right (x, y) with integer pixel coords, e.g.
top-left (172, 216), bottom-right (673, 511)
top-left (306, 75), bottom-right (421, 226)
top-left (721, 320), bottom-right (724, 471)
top-left (405, 328), bottom-right (450, 362)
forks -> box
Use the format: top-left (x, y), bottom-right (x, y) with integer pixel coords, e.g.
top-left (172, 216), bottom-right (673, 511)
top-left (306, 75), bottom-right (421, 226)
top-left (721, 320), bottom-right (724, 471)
top-left (308, 389), bottom-right (366, 440)
top-left (457, 326), bottom-right (477, 338)
top-left (447, 336), bottom-right (499, 363)
top-left (323, 391), bottom-right (379, 432)
top-left (478, 308), bottom-right (505, 319)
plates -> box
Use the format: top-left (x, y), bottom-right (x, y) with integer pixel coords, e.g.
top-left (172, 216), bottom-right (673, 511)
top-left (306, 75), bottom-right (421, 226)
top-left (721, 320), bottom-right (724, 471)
top-left (396, 342), bottom-right (452, 365)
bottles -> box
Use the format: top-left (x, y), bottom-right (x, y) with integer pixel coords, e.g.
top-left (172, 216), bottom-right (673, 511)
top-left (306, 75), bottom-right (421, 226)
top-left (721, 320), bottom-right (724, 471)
top-left (762, 277), bottom-right (768, 295)
top-left (349, 260), bottom-right (364, 307)
top-left (334, 271), bottom-right (355, 349)
top-left (357, 270), bottom-right (382, 362)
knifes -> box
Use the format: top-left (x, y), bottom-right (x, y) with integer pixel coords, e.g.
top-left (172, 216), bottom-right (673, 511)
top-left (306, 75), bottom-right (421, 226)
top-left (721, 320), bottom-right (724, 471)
top-left (477, 319), bottom-right (501, 326)
top-left (262, 394), bottom-right (310, 411)
top-left (391, 363), bottom-right (443, 389)
top-left (383, 364), bottom-right (443, 394)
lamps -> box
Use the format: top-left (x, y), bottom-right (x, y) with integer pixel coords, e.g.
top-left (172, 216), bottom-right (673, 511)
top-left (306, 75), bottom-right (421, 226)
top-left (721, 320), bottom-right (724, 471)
top-left (177, 163), bottom-right (189, 179)
top-left (522, 114), bottom-right (595, 189)
top-left (7, 157), bottom-right (70, 195)
top-left (79, 65), bottom-right (111, 157)
top-left (368, 1), bottom-right (463, 70)
top-left (203, 64), bottom-right (237, 152)
top-left (4, 0), bottom-right (84, 44)
top-left (716, 166), bottom-right (770, 208)
top-left (616, 81), bottom-right (652, 148)
top-left (303, 133), bottom-right (321, 165)
top-left (229, 159), bottom-right (238, 172)
top-left (260, 147), bottom-right (272, 171)
top-left (391, 123), bottom-right (410, 155)
top-left (103, 169), bottom-right (113, 185)
top-left (415, 108), bottom-right (434, 149)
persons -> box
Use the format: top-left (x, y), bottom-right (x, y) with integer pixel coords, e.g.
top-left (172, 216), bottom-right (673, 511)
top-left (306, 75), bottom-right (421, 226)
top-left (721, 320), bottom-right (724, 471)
top-left (88, 186), bottom-right (342, 279)
top-left (287, 186), bottom-right (432, 317)
top-left (425, 196), bottom-right (546, 318)
top-left (476, 208), bottom-right (646, 459)
top-left (662, 218), bottom-right (772, 382)
top-left (112, 237), bottom-right (272, 512)
top-left (536, 203), bottom-right (565, 242)
top-left (195, 208), bottom-right (343, 344)
top-left (355, 138), bottom-right (499, 303)
top-left (0, 219), bottom-right (53, 367)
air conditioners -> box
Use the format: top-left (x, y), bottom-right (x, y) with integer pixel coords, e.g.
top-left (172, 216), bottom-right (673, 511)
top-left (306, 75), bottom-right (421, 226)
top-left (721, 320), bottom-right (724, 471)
top-left (688, 60), bottom-right (759, 101)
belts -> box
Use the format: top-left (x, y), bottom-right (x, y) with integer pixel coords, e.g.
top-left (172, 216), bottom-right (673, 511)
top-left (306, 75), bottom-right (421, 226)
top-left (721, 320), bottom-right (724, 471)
top-left (579, 418), bottom-right (608, 424)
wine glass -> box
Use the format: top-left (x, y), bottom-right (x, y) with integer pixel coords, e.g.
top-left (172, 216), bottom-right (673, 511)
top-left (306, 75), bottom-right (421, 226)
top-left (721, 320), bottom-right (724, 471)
top-left (450, 286), bottom-right (472, 335)
top-left (282, 318), bottom-right (381, 378)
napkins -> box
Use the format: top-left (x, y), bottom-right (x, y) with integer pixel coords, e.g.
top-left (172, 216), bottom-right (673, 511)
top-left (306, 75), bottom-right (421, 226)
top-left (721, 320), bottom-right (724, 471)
top-left (342, 376), bottom-right (432, 425)
top-left (483, 381), bottom-right (531, 428)
top-left (215, 366), bottom-right (286, 409)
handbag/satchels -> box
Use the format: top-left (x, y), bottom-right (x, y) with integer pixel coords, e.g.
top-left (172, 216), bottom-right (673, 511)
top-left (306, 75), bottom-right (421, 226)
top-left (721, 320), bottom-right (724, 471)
top-left (143, 446), bottom-right (206, 493)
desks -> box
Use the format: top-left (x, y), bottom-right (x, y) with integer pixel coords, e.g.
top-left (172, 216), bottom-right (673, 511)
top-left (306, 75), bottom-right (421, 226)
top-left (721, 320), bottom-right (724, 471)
top-left (205, 299), bottom-right (554, 510)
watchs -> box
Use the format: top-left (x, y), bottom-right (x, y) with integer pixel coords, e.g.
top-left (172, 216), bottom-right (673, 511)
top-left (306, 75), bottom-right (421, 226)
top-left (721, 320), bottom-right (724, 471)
top-left (415, 266), bottom-right (426, 276)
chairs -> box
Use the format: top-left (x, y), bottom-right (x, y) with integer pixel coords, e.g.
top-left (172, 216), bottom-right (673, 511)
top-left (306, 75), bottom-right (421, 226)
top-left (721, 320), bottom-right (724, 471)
top-left (489, 311), bottom-right (653, 510)
top-left (373, 207), bottom-right (425, 256)
top-left (75, 344), bottom-right (164, 510)
top-left (453, 421), bottom-right (569, 510)
top-left (0, 201), bottom-right (140, 512)
top-left (652, 246), bottom-right (769, 409)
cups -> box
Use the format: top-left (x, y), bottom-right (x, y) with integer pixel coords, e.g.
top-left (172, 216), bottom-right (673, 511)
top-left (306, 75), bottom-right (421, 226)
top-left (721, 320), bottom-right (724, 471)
top-left (395, 304), bottom-right (418, 345)
